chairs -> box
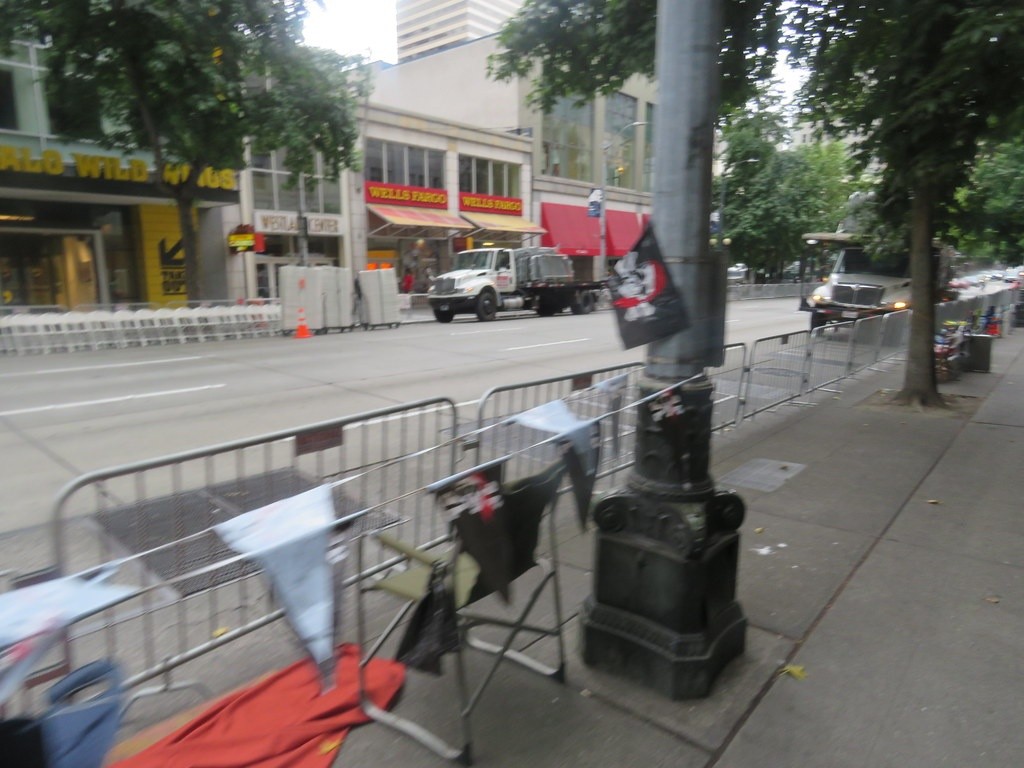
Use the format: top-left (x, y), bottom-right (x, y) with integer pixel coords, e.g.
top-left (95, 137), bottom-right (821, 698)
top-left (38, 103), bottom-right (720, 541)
top-left (357, 457), bottom-right (569, 761)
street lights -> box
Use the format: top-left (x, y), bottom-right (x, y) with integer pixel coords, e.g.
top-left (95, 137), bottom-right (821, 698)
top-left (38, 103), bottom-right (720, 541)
top-left (717, 158), bottom-right (760, 250)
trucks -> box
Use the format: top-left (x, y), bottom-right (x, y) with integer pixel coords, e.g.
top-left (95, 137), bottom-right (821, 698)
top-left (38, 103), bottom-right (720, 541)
top-left (426, 247), bottom-right (611, 324)
top-left (795, 192), bottom-right (961, 335)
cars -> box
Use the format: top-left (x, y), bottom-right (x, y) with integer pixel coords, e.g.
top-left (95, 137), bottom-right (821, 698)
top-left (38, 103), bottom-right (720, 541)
top-left (946, 264), bottom-right (1024, 302)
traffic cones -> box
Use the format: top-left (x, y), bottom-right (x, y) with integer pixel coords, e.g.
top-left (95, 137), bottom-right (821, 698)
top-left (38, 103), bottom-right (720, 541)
top-left (292, 306), bottom-right (315, 338)
